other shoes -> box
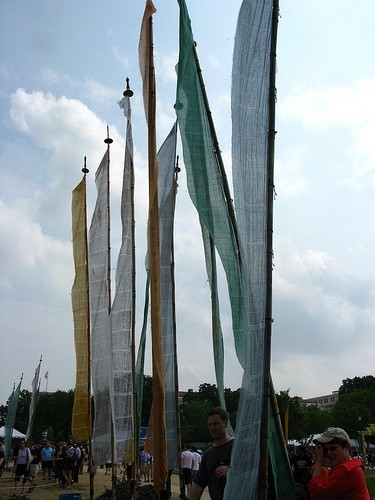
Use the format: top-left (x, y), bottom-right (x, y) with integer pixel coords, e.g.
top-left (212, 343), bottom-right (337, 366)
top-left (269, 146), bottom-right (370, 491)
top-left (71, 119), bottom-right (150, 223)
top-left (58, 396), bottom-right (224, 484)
top-left (11, 492), bottom-right (16, 497)
top-left (20, 492), bottom-right (24, 496)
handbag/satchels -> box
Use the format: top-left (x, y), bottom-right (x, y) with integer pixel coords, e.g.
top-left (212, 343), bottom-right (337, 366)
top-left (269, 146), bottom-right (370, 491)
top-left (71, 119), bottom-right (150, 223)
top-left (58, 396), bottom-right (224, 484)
top-left (26, 448), bottom-right (34, 462)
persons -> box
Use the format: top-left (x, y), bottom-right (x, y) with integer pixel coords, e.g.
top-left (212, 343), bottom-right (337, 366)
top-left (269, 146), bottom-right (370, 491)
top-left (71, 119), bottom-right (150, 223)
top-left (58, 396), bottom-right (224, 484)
top-left (0, 438), bottom-right (86, 497)
top-left (190, 407), bottom-right (251, 500)
top-left (353, 451), bottom-right (366, 471)
top-left (124, 447), bottom-right (152, 483)
top-left (105, 453), bottom-right (112, 476)
top-left (308, 427), bottom-right (371, 500)
top-left (180, 443), bottom-right (201, 497)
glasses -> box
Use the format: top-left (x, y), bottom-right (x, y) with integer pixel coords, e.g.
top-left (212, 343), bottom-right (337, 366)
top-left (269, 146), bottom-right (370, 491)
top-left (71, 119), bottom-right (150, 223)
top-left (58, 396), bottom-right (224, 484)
top-left (323, 445), bottom-right (341, 453)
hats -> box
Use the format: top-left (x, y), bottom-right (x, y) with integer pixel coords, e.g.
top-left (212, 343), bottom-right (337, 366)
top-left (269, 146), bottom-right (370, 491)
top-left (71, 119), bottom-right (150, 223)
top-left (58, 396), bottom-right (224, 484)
top-left (313, 427), bottom-right (352, 448)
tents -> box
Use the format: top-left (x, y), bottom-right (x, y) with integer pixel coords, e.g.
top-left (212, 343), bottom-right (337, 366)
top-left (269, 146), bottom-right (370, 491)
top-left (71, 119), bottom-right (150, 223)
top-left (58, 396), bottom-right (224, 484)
top-left (286, 431), bottom-right (375, 449)
top-left (0, 426), bottom-right (28, 438)
